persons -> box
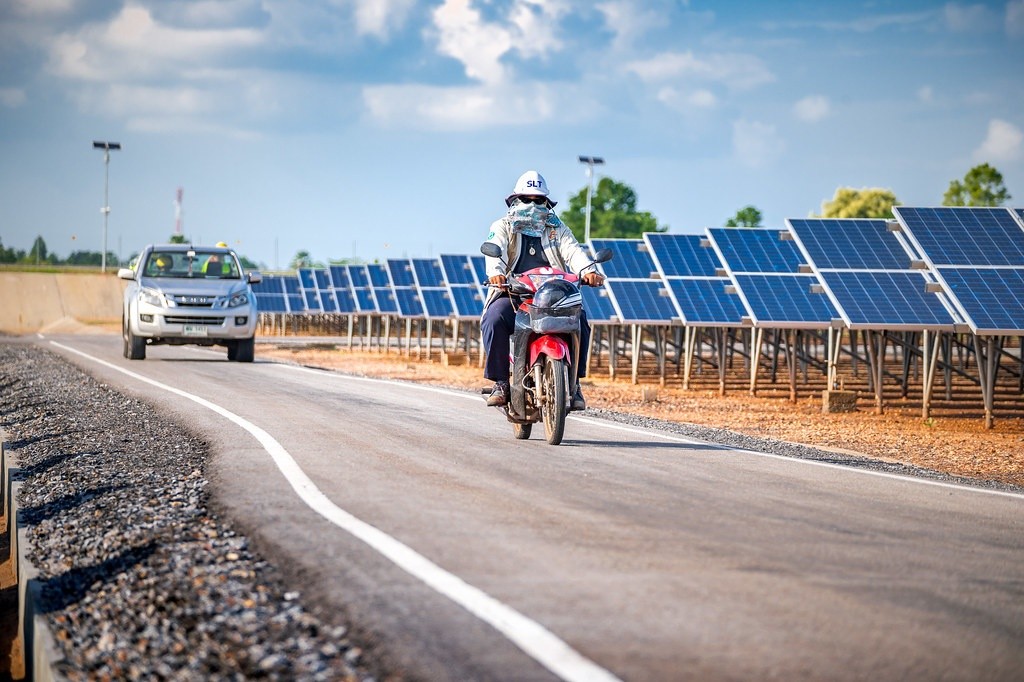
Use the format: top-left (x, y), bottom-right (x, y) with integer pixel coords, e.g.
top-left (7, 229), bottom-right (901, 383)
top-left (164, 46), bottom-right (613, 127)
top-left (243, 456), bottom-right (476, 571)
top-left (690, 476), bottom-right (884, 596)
top-left (202, 242), bottom-right (230, 274)
top-left (156, 255), bottom-right (173, 272)
top-left (480, 170), bottom-right (607, 410)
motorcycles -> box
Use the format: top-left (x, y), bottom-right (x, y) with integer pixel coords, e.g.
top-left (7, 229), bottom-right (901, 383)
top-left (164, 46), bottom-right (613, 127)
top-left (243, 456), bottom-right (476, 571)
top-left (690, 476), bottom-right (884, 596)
top-left (480, 241), bottom-right (613, 446)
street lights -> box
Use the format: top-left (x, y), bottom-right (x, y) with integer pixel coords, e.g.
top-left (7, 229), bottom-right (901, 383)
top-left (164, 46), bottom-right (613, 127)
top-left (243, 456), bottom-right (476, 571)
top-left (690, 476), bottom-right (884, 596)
top-left (578, 154), bottom-right (606, 242)
top-left (91, 138), bottom-right (122, 273)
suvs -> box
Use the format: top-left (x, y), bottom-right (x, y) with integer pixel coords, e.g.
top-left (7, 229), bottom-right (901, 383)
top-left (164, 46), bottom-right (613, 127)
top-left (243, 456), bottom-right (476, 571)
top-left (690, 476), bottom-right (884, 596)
top-left (115, 243), bottom-right (262, 363)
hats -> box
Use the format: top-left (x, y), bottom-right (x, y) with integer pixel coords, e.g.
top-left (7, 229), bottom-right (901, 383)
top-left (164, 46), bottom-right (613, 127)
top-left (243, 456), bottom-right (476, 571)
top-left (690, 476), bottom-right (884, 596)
top-left (505, 171), bottom-right (558, 210)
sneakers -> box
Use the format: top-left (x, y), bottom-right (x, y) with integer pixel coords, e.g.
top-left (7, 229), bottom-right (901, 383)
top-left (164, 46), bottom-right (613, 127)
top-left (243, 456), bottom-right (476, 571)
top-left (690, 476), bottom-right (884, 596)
top-left (486, 382), bottom-right (510, 407)
top-left (571, 385), bottom-right (585, 410)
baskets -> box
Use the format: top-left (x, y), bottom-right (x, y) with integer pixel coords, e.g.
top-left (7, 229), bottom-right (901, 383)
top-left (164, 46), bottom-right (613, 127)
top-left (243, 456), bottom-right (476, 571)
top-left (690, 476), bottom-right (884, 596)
top-left (527, 304), bottom-right (582, 334)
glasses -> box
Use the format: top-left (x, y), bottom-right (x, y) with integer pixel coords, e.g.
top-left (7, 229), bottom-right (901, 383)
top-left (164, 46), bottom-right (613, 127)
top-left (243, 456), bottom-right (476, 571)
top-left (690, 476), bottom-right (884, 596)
top-left (517, 195), bottom-right (547, 205)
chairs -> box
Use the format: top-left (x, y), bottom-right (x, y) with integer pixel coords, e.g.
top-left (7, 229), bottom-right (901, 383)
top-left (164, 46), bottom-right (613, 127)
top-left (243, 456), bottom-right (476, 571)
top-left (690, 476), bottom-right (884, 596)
top-left (203, 255), bottom-right (230, 277)
top-left (154, 254), bottom-right (173, 277)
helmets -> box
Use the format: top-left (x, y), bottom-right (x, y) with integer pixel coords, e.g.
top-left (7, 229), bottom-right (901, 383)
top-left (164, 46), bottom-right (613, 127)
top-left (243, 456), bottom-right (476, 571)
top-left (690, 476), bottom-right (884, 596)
top-left (532, 279), bottom-right (582, 316)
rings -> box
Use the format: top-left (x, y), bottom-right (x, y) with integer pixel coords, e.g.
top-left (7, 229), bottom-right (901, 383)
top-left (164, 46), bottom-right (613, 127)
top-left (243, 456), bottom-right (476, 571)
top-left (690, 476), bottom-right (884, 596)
top-left (596, 282), bottom-right (600, 285)
